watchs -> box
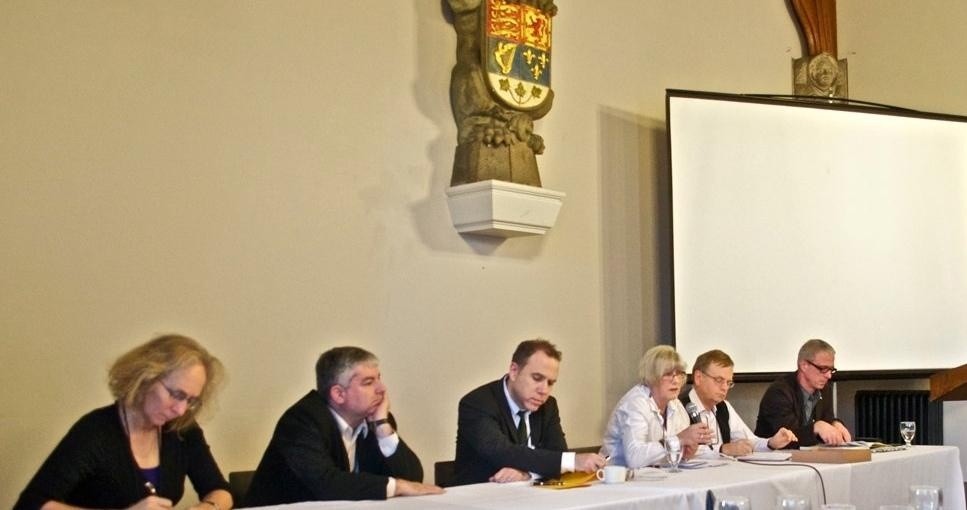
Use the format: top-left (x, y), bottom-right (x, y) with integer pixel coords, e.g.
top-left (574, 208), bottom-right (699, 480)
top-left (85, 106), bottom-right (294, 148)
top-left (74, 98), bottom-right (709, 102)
top-left (200, 501), bottom-right (221, 510)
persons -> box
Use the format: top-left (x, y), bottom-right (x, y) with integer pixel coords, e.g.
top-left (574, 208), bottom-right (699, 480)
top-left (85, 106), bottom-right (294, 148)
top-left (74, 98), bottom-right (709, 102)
top-left (454, 338), bottom-right (606, 483)
top-left (242, 344), bottom-right (447, 507)
top-left (12, 331), bottom-right (237, 510)
top-left (598, 344), bottom-right (716, 469)
top-left (753, 339), bottom-right (852, 450)
top-left (676, 348), bottom-right (798, 457)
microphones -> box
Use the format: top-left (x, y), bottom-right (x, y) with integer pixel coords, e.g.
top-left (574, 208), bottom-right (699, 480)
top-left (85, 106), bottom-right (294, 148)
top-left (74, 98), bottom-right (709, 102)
top-left (686, 402), bottom-right (718, 450)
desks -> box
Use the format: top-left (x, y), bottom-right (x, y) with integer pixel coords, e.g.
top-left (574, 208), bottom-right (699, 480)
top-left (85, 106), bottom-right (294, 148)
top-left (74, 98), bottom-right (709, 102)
top-left (244, 445), bottom-right (966, 510)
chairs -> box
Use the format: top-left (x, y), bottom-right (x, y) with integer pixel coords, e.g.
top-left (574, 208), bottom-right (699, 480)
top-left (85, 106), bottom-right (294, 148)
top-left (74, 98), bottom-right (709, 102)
top-left (435, 460), bottom-right (458, 487)
top-left (229, 470), bottom-right (254, 508)
top-left (566, 446), bottom-right (601, 455)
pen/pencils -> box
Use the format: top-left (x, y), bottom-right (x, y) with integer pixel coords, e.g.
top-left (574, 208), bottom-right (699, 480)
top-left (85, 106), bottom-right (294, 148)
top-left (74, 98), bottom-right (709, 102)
top-left (144, 481), bottom-right (157, 496)
top-left (647, 465), bottom-right (660, 468)
top-left (533, 481), bottom-right (570, 486)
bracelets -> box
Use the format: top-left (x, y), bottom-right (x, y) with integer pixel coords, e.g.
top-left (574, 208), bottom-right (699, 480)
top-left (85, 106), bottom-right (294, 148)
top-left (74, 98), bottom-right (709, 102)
top-left (367, 418), bottom-right (394, 433)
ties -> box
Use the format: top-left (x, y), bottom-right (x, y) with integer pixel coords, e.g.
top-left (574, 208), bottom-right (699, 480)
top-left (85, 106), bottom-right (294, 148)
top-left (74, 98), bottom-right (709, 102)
top-left (514, 409), bottom-right (530, 447)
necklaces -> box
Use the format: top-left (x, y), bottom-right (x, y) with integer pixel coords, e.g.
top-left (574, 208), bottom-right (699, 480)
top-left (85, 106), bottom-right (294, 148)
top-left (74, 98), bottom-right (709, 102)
top-left (121, 398), bottom-right (163, 500)
top-left (648, 390), bottom-right (666, 411)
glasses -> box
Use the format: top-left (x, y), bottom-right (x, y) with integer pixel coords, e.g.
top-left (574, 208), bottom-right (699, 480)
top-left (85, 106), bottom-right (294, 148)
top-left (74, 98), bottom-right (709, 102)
top-left (659, 372), bottom-right (686, 382)
top-left (806, 360), bottom-right (838, 374)
top-left (157, 376), bottom-right (201, 412)
top-left (699, 369), bottom-right (735, 390)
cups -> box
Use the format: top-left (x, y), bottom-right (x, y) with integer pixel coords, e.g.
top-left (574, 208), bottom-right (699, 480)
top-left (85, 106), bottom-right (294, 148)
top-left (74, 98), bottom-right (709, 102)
top-left (596, 465), bottom-right (627, 483)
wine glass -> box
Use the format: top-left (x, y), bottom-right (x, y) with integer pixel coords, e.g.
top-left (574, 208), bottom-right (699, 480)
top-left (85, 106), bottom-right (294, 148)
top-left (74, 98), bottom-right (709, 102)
top-left (664, 421), bottom-right (943, 510)
